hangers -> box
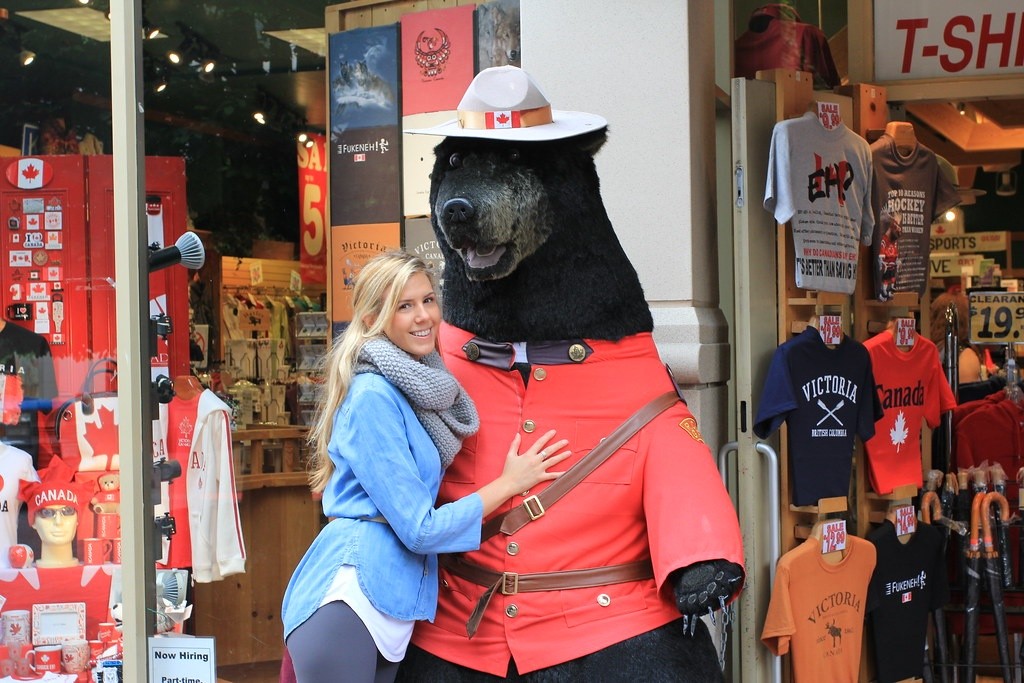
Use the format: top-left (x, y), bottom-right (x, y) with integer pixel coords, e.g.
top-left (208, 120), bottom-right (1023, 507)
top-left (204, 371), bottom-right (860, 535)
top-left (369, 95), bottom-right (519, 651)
top-left (809, 313), bottom-right (844, 341)
top-left (886, 318), bottom-right (917, 340)
top-left (224, 284), bottom-right (297, 303)
top-left (809, 101), bottom-right (840, 127)
top-left (885, 121), bottom-right (916, 149)
top-left (885, 505), bottom-right (917, 537)
top-left (811, 518), bottom-right (851, 555)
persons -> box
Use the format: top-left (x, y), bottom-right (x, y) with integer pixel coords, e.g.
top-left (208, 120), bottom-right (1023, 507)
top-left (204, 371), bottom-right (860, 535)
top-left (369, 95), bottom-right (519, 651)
top-left (280, 250), bottom-right (571, 683)
top-left (929, 292), bottom-right (980, 384)
top-left (27, 478), bottom-right (84, 568)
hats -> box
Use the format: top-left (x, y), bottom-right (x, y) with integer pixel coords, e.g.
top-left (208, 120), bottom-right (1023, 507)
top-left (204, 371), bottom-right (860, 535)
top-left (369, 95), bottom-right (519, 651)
top-left (17, 452), bottom-right (94, 526)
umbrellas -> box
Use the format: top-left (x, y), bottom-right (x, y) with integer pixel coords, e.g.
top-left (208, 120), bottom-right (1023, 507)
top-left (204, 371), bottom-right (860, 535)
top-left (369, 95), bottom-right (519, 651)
top-left (920, 459), bottom-right (1024, 683)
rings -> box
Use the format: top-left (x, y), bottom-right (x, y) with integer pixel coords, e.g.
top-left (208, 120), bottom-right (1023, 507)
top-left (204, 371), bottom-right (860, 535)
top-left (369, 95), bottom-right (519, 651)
top-left (541, 450), bottom-right (547, 459)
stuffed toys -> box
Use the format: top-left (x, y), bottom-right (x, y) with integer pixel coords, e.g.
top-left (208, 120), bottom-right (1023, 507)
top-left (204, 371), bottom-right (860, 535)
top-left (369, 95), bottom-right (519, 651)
top-left (395, 65), bottom-right (747, 683)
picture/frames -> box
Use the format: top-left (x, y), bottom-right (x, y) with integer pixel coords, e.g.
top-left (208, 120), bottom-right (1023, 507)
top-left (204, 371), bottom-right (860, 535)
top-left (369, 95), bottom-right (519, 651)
top-left (31, 602), bottom-right (87, 645)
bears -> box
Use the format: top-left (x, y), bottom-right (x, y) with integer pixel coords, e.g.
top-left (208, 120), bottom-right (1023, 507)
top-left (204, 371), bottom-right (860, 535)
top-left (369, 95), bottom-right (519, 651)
top-left (392, 127), bottom-right (745, 683)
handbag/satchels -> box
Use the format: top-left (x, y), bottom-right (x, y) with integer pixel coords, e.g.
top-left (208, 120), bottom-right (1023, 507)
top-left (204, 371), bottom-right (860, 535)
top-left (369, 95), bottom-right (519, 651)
top-left (54, 357), bottom-right (118, 472)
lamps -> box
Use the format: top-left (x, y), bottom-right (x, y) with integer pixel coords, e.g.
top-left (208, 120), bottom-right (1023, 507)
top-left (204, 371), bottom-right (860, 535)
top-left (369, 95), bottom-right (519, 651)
top-left (147, 230), bottom-right (205, 271)
top-left (958, 103), bottom-right (966, 115)
top-left (152, 34), bottom-right (220, 97)
top-left (156, 568), bottom-right (187, 635)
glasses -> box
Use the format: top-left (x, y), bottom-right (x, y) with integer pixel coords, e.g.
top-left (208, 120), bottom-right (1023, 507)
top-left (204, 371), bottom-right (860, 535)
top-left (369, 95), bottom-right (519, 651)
top-left (36, 505), bottom-right (76, 518)
